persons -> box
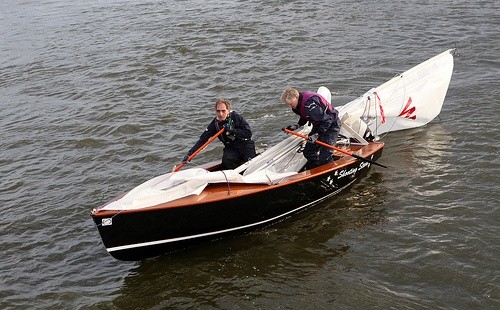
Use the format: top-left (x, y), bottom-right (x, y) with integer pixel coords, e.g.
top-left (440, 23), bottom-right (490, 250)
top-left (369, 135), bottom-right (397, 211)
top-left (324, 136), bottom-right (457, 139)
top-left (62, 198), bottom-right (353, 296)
top-left (281, 88), bottom-right (342, 170)
top-left (182, 97), bottom-right (256, 171)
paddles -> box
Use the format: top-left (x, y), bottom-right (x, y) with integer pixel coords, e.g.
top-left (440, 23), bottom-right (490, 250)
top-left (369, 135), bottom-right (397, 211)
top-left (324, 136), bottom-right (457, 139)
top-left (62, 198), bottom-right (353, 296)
top-left (283, 129), bottom-right (388, 169)
top-left (171, 126), bottom-right (226, 173)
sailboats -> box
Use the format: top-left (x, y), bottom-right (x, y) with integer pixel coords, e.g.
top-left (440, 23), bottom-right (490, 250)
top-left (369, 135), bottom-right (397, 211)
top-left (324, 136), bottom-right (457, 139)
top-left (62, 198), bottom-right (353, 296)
top-left (89, 46), bottom-right (456, 261)
